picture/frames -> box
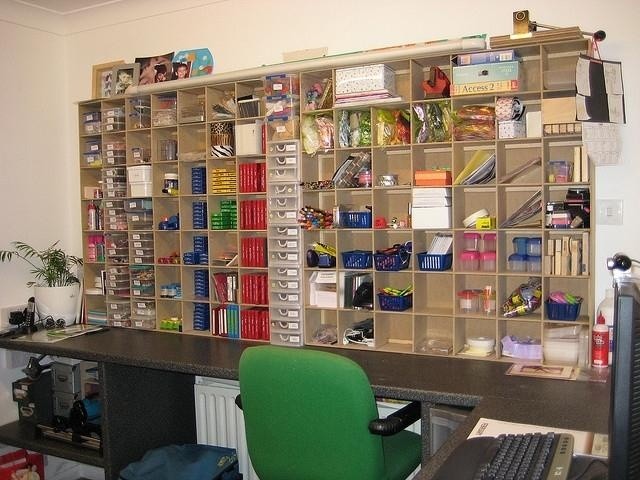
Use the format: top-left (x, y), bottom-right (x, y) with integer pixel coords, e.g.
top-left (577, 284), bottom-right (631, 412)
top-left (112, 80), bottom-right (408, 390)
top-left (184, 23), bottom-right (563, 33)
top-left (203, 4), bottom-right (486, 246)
top-left (91, 60), bottom-right (126, 99)
top-left (111, 62), bottom-right (141, 97)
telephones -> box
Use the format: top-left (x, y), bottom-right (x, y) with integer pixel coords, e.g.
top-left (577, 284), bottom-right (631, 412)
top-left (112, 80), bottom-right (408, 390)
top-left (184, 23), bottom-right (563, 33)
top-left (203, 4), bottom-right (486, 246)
top-left (22, 296), bottom-right (38, 334)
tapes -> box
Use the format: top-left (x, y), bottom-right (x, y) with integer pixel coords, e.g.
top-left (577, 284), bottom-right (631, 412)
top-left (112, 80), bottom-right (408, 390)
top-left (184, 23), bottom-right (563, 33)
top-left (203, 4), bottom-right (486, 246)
top-left (466, 336), bottom-right (495, 347)
top-left (462, 208), bottom-right (490, 228)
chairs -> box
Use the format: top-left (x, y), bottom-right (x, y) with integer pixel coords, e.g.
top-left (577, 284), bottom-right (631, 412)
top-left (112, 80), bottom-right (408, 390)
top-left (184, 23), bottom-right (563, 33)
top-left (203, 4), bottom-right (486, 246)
top-left (234, 345), bottom-right (423, 480)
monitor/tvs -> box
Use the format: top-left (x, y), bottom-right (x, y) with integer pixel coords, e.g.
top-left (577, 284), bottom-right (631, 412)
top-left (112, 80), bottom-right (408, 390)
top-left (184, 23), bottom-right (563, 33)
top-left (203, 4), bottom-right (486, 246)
top-left (607, 263), bottom-right (640, 480)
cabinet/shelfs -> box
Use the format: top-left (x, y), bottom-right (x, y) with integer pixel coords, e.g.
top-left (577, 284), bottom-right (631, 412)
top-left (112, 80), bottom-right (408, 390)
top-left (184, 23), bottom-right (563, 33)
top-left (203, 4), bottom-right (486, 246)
top-left (298, 37), bottom-right (596, 371)
top-left (74, 62), bottom-right (304, 345)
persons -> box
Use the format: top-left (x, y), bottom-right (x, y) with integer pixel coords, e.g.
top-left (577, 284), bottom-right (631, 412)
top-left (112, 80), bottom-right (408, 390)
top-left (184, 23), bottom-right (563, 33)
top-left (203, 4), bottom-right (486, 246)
top-left (115, 52), bottom-right (188, 95)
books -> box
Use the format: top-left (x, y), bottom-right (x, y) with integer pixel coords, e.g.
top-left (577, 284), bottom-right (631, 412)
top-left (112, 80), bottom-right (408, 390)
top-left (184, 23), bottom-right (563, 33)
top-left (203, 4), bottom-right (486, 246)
top-left (334, 88), bottom-right (404, 109)
top-left (544, 124), bottom-right (590, 183)
top-left (544, 231), bottom-right (590, 276)
top-left (211, 162), bottom-right (271, 341)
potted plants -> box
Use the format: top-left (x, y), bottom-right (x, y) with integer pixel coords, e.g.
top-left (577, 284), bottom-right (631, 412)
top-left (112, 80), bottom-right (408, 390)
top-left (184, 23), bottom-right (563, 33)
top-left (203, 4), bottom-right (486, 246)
top-left (0, 237), bottom-right (84, 327)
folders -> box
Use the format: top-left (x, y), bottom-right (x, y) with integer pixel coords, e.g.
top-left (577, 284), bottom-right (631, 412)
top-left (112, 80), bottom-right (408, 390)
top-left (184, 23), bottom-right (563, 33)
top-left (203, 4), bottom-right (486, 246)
top-left (457, 49), bottom-right (521, 66)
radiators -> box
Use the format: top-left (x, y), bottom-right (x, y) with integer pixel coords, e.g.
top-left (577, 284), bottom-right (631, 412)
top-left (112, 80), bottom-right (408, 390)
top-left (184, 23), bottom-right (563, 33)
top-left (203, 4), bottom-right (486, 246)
top-left (194, 375), bottom-right (422, 480)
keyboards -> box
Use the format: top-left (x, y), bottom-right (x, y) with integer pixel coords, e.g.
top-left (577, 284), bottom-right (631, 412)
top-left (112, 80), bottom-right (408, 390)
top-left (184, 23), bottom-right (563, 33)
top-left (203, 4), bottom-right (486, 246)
top-left (475, 432), bottom-right (575, 480)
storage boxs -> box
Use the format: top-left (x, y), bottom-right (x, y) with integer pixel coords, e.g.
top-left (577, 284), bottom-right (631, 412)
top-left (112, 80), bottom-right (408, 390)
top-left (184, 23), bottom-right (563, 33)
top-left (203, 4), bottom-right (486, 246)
top-left (84, 95), bottom-right (156, 328)
top-left (451, 60), bottom-right (521, 83)
top-left (263, 73), bottom-right (302, 350)
top-left (450, 50), bottom-right (515, 65)
top-left (335, 64), bottom-right (397, 99)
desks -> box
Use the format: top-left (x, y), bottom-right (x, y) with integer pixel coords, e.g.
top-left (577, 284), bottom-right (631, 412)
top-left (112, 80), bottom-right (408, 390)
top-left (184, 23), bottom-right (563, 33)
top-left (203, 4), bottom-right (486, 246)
top-left (0, 325), bottom-right (613, 480)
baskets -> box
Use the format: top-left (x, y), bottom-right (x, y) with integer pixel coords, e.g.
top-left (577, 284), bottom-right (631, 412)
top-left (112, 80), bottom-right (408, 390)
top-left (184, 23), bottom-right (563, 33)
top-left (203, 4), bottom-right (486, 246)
top-left (340, 247), bottom-right (372, 269)
top-left (416, 250), bottom-right (453, 272)
top-left (342, 209), bottom-right (372, 229)
top-left (374, 249), bottom-right (411, 272)
top-left (377, 289), bottom-right (412, 311)
top-left (546, 295), bottom-right (584, 321)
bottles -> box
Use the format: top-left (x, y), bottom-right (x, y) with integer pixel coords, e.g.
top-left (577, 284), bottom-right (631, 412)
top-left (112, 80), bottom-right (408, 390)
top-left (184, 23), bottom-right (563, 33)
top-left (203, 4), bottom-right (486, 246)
top-left (592, 311), bottom-right (609, 370)
